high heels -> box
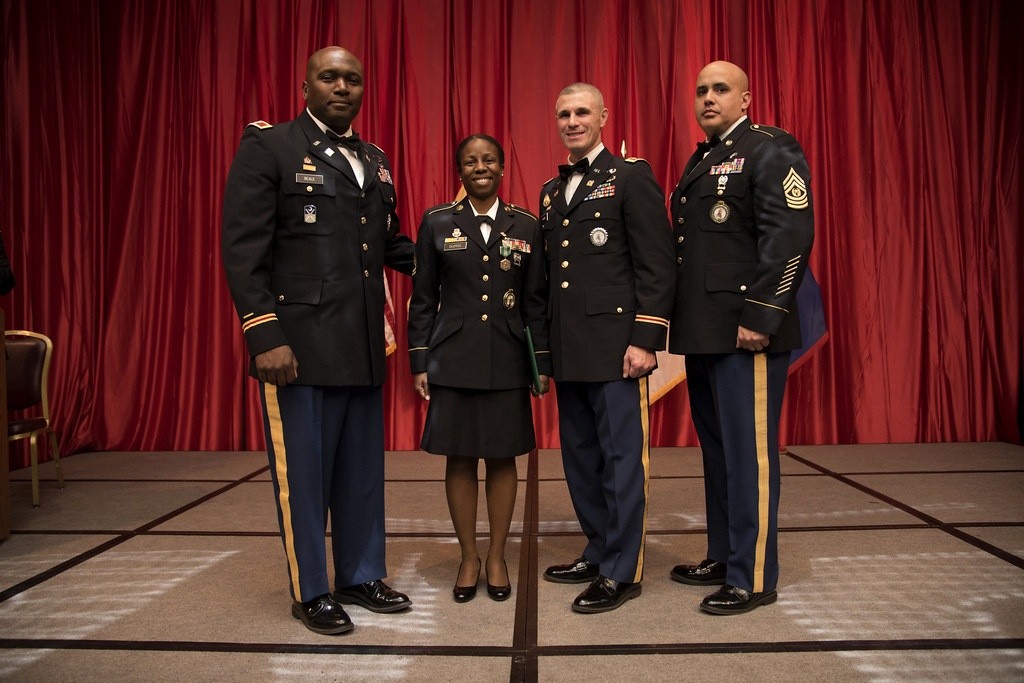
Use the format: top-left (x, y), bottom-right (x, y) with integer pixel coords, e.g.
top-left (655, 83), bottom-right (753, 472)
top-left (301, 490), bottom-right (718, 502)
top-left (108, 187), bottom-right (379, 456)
top-left (484, 556), bottom-right (512, 601)
top-left (453, 554), bottom-right (481, 602)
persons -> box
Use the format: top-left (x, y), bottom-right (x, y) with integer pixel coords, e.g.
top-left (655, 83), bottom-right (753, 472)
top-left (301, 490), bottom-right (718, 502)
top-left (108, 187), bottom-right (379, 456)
top-left (221, 46), bottom-right (424, 635)
top-left (539, 82), bottom-right (671, 614)
top-left (669, 61), bottom-right (814, 613)
top-left (407, 134), bottom-right (549, 601)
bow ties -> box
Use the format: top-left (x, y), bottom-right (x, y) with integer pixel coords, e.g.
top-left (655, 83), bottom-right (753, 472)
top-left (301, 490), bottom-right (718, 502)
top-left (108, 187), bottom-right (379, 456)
top-left (474, 215), bottom-right (495, 227)
top-left (694, 136), bottom-right (723, 151)
top-left (325, 129), bottom-right (361, 150)
top-left (558, 157), bottom-right (589, 176)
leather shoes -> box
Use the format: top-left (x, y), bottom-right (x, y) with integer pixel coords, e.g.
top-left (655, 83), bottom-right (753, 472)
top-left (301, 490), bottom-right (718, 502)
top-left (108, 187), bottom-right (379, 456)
top-left (671, 559), bottom-right (777, 616)
top-left (542, 559), bottom-right (643, 614)
top-left (292, 595), bottom-right (354, 636)
top-left (333, 579), bottom-right (412, 613)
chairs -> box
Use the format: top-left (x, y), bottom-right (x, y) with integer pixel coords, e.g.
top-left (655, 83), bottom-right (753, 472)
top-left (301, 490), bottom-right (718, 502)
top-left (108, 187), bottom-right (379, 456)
top-left (4, 329), bottom-right (67, 509)
